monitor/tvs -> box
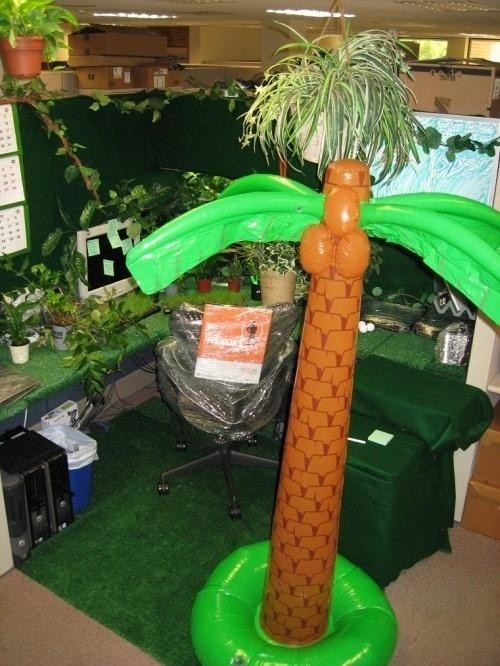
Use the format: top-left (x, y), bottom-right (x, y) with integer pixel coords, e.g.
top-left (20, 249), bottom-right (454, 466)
top-left (76, 218), bottom-right (140, 302)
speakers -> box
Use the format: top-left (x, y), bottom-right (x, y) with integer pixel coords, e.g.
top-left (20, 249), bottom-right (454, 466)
top-left (0, 426), bottom-right (76, 563)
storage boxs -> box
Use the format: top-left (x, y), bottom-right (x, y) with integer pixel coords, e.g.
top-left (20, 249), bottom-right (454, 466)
top-left (398, 55), bottom-right (499, 117)
top-left (66, 23), bottom-right (186, 88)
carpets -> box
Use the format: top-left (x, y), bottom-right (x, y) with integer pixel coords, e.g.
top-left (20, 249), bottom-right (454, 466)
top-left (15, 395), bottom-right (283, 666)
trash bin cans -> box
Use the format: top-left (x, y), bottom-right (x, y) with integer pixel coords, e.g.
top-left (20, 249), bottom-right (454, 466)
top-left (30, 419), bottom-right (98, 515)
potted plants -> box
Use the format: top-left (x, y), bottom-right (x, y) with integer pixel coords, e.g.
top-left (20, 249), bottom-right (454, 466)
top-left (236, 17), bottom-right (429, 188)
top-left (0, 286), bottom-right (81, 363)
top-left (196, 242), bottom-right (300, 306)
top-left (0, 0), bottom-right (81, 78)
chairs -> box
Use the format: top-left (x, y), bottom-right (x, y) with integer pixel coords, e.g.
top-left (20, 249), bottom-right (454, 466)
top-left (152, 306), bottom-right (304, 522)
